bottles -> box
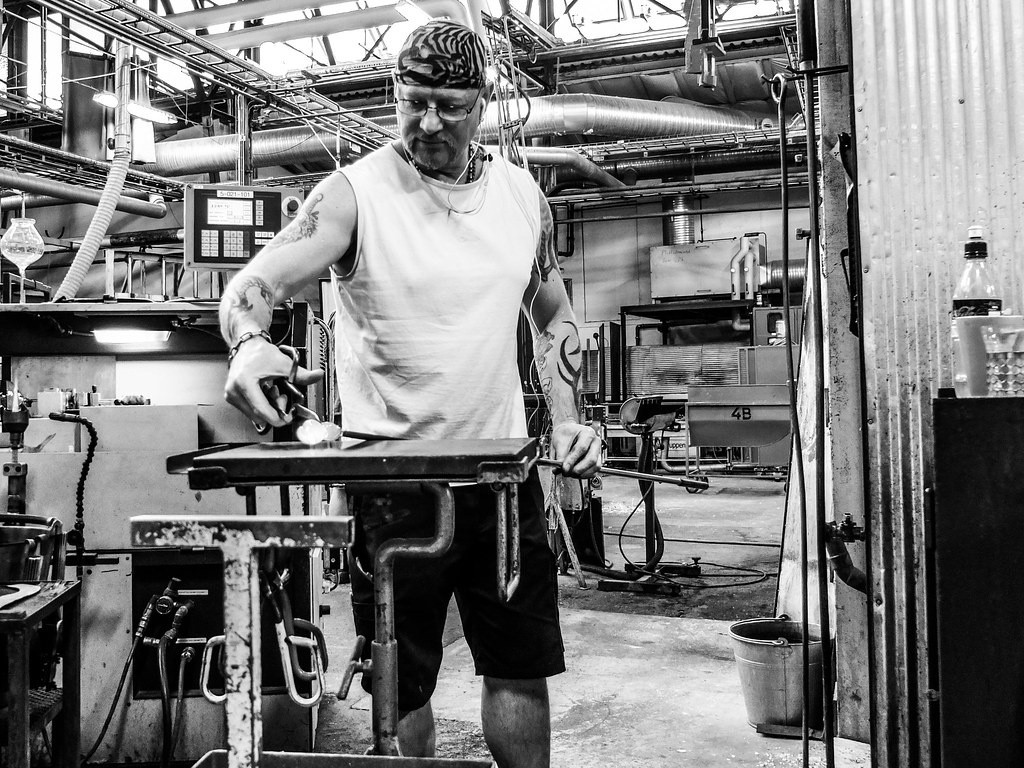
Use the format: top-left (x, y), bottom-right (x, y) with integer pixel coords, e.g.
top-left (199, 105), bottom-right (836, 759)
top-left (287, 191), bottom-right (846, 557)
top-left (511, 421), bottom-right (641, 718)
top-left (950, 225), bottom-right (1003, 398)
top-left (776, 320), bottom-right (785, 335)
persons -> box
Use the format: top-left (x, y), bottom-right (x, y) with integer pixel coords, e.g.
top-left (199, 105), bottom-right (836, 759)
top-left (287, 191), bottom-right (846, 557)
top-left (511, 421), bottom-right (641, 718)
top-left (219, 23), bottom-right (603, 768)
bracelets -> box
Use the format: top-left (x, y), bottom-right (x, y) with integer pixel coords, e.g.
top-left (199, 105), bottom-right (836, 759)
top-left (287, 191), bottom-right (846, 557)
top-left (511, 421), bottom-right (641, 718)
top-left (228, 330), bottom-right (271, 368)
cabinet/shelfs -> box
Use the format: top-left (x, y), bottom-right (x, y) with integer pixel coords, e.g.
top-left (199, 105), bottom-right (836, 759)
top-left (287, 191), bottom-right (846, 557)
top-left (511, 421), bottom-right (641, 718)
top-left (0, 579), bottom-right (83, 768)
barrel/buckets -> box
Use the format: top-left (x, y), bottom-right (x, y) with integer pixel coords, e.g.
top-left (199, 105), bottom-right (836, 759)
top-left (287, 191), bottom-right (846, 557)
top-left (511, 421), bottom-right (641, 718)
top-left (727, 613), bottom-right (837, 730)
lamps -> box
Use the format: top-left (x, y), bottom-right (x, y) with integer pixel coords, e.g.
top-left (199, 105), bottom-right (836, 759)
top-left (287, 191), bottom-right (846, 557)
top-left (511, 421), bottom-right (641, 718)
top-left (393, 0), bottom-right (514, 92)
top-left (91, 89), bottom-right (178, 125)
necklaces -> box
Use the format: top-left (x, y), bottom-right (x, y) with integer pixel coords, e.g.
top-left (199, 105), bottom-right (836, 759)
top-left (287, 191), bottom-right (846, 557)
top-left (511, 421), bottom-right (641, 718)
top-left (403, 145), bottom-right (476, 184)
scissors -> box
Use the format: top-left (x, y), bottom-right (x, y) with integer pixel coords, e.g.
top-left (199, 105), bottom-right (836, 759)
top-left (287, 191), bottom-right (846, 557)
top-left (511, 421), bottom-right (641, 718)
top-left (252, 343), bottom-right (321, 435)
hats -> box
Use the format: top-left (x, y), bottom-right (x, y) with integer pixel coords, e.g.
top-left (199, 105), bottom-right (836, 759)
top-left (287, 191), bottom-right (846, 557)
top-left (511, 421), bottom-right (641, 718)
top-left (393, 19), bottom-right (488, 90)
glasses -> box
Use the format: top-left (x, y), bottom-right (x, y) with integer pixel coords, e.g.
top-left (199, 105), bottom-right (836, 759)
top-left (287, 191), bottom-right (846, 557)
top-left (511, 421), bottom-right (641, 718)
top-left (393, 77), bottom-right (483, 122)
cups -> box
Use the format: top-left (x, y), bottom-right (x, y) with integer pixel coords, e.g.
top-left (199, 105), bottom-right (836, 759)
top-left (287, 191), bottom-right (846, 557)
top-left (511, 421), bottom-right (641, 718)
top-left (957, 316), bottom-right (1024, 396)
top-left (980, 325), bottom-right (1024, 397)
